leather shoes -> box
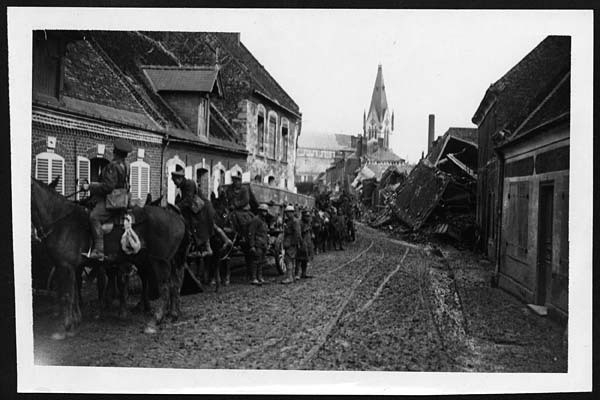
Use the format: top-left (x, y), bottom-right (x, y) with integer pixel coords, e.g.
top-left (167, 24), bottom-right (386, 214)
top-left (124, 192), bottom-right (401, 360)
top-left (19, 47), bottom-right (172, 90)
top-left (263, 280), bottom-right (269, 284)
top-left (251, 280), bottom-right (262, 287)
top-left (80, 250), bottom-right (105, 262)
top-left (279, 278), bottom-right (293, 284)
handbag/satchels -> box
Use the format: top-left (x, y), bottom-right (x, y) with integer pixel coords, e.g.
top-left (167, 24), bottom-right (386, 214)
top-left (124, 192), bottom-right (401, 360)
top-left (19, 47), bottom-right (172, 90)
top-left (104, 188), bottom-right (129, 208)
top-left (190, 194), bottom-right (205, 213)
top-left (237, 210), bottom-right (252, 225)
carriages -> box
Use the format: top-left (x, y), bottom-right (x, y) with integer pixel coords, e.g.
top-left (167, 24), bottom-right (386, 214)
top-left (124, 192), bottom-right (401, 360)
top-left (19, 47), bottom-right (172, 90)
top-left (328, 206), bottom-right (357, 242)
top-left (208, 192), bottom-right (292, 291)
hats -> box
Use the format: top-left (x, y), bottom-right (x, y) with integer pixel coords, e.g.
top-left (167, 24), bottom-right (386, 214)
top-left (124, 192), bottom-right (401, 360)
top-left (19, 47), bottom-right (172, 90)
top-left (171, 168), bottom-right (186, 177)
top-left (114, 139), bottom-right (133, 155)
top-left (301, 210), bottom-right (312, 216)
top-left (258, 204), bottom-right (270, 211)
top-left (284, 205), bottom-right (294, 211)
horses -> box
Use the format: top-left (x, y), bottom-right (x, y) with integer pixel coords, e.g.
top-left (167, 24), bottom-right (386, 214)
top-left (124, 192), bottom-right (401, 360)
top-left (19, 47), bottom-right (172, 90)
top-left (32, 178), bottom-right (189, 341)
top-left (143, 172), bottom-right (355, 291)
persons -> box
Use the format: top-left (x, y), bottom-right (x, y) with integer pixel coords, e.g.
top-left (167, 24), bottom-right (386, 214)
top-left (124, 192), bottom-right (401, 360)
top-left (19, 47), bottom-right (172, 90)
top-left (171, 170), bottom-right (216, 258)
top-left (80, 138), bottom-right (132, 261)
top-left (226, 171), bottom-right (349, 286)
